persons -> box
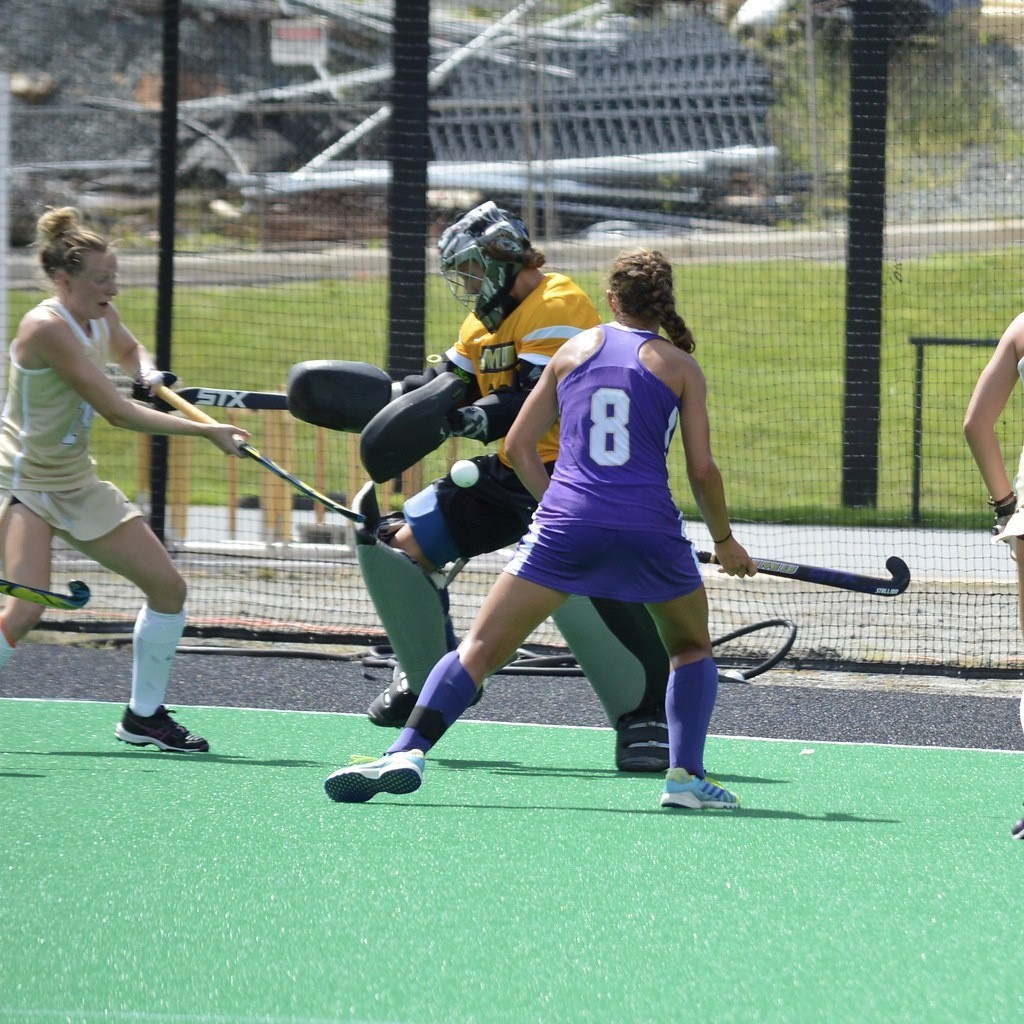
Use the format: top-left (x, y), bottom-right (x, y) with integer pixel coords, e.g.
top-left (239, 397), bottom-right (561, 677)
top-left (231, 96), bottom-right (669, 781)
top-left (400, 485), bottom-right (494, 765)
top-left (287, 201), bottom-right (670, 769)
top-left (962, 312), bottom-right (1024, 838)
top-left (0, 207), bottom-right (253, 751)
top-left (323, 248), bottom-right (757, 810)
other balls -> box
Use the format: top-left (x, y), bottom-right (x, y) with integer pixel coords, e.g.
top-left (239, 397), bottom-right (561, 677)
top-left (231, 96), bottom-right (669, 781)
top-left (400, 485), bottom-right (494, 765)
top-left (450, 459), bottom-right (481, 488)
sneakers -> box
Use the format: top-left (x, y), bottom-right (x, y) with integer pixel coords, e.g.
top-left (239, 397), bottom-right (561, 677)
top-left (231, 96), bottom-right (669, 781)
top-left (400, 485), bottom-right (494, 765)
top-left (1011, 816), bottom-right (1024, 839)
top-left (323, 748), bottom-right (425, 803)
top-left (660, 767), bottom-right (741, 809)
top-left (114, 704), bottom-right (210, 753)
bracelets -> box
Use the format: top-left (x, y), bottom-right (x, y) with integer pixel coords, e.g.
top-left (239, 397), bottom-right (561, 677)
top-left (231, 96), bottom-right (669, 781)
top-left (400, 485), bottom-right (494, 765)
top-left (988, 489), bottom-right (1018, 517)
top-left (714, 530), bottom-right (731, 543)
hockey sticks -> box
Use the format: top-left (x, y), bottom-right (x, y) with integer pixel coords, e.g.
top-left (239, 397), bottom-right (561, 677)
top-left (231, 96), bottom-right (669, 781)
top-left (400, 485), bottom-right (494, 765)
top-left (116, 371), bottom-right (290, 413)
top-left (156, 380), bottom-right (368, 527)
top-left (0, 577), bottom-right (91, 611)
top-left (694, 548), bottom-right (911, 598)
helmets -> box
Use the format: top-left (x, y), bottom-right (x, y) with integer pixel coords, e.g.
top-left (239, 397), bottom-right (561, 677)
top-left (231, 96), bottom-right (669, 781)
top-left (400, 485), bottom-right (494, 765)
top-left (437, 199), bottom-right (535, 335)
